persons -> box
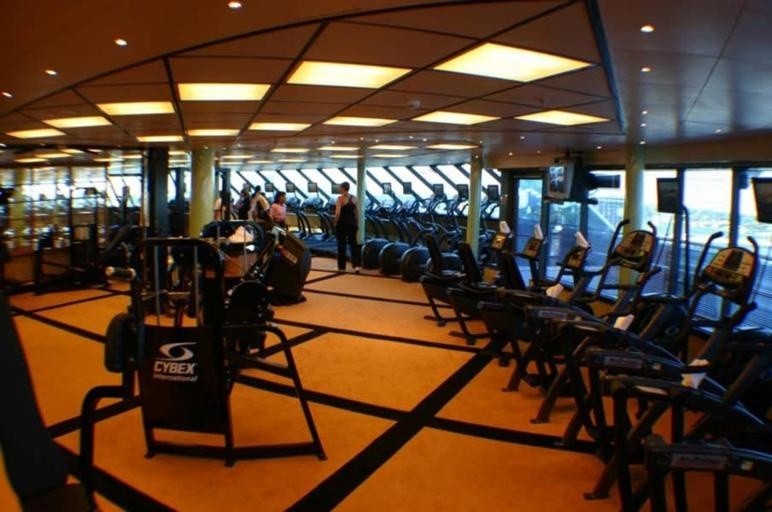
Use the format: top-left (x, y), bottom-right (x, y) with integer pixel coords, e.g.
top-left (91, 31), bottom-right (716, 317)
top-left (332, 180), bottom-right (361, 274)
top-left (213, 191), bottom-right (226, 220)
top-left (236, 186), bottom-right (269, 220)
top-left (268, 192), bottom-right (287, 229)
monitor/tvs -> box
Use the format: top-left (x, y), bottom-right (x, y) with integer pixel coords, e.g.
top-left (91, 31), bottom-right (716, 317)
top-left (542, 161), bottom-right (598, 206)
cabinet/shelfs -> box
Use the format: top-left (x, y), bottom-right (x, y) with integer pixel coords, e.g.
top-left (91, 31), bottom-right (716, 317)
top-left (0, 190), bottom-right (108, 282)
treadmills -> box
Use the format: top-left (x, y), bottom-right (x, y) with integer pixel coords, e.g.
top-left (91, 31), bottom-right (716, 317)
top-left (282, 193), bottom-right (356, 259)
